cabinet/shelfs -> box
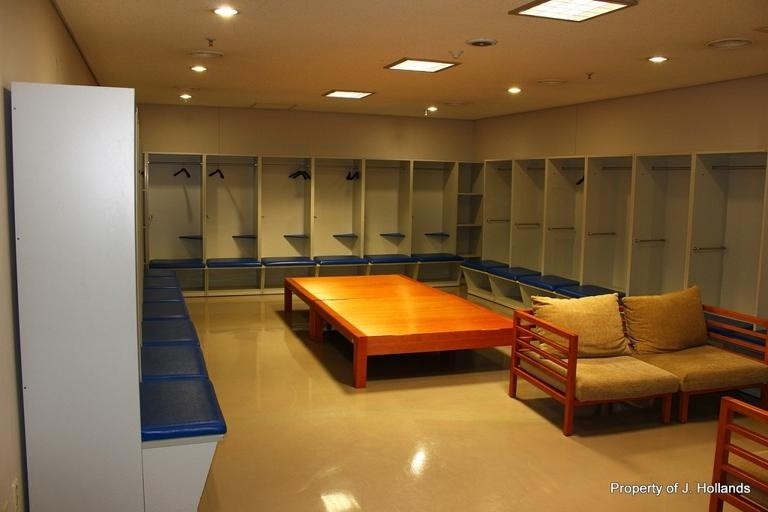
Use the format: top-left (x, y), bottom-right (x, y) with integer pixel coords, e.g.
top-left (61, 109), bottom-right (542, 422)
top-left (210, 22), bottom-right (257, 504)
top-left (9, 82), bottom-right (768, 512)
